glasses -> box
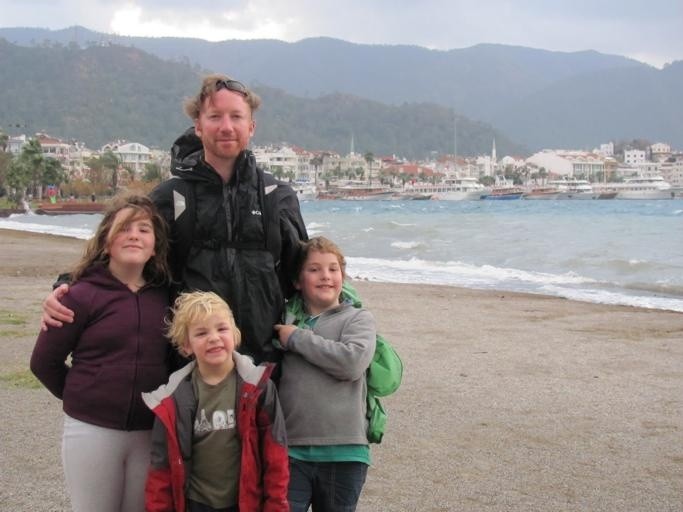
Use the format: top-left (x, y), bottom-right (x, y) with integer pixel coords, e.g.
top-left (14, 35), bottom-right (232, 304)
top-left (200, 80), bottom-right (248, 100)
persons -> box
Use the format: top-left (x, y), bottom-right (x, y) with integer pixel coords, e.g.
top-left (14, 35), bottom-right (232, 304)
top-left (141, 290), bottom-right (291, 512)
top-left (273, 237), bottom-right (377, 512)
top-left (39, 75), bottom-right (308, 393)
top-left (30, 195), bottom-right (180, 512)
